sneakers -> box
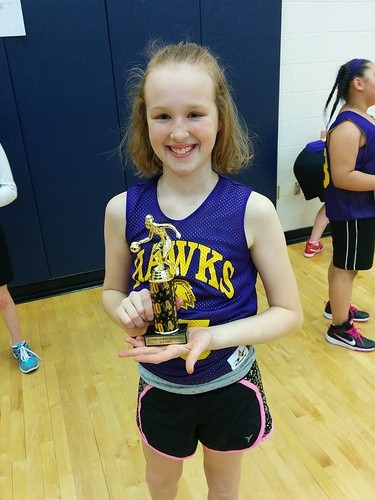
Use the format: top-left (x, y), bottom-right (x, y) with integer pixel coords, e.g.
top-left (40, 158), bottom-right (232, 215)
top-left (303, 238), bottom-right (324, 258)
top-left (324, 300), bottom-right (369, 321)
top-left (12, 340), bottom-right (39, 374)
top-left (326, 315), bottom-right (375, 351)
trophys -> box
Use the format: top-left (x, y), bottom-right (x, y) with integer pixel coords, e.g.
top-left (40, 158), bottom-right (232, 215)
top-left (130, 214), bottom-right (188, 347)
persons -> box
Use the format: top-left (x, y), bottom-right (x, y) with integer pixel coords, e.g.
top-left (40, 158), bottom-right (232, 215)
top-left (293, 136), bottom-right (331, 258)
top-left (100, 41), bottom-right (304, 500)
top-left (323, 57), bottom-right (375, 352)
top-left (0, 139), bottom-right (43, 374)
